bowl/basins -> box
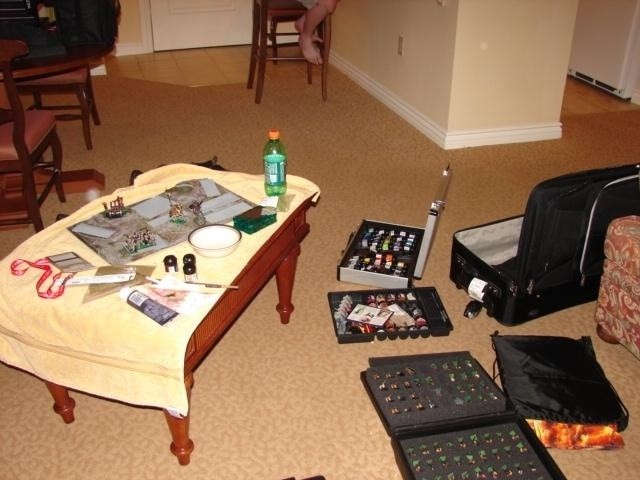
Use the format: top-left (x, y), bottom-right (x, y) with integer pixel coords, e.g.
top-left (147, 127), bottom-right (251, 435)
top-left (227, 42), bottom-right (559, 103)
top-left (187, 223), bottom-right (242, 260)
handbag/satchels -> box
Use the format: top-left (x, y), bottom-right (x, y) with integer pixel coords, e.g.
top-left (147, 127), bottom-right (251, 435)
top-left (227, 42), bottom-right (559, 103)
top-left (488, 332), bottom-right (629, 435)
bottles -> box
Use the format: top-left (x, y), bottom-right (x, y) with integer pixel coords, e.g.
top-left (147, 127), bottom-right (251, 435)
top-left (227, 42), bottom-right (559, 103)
top-left (182, 254), bottom-right (198, 282)
top-left (349, 229), bottom-right (418, 277)
top-left (366, 292), bottom-right (430, 333)
top-left (163, 255), bottom-right (178, 274)
top-left (263, 128), bottom-right (287, 196)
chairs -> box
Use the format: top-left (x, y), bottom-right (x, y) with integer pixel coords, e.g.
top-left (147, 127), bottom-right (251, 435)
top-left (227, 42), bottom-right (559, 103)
top-left (1, 37), bottom-right (109, 152)
top-left (1, 110), bottom-right (66, 232)
top-left (247, 0), bottom-right (332, 104)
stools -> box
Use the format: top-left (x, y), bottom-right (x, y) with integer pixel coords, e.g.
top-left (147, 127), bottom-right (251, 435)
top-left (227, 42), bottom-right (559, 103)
top-left (597, 214), bottom-right (640, 358)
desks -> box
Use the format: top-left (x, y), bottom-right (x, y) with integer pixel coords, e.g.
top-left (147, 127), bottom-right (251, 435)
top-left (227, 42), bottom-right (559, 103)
top-left (0, 161), bottom-right (318, 465)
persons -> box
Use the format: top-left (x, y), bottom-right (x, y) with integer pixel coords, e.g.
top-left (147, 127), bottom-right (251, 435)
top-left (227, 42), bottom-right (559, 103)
top-left (294, 0), bottom-right (340, 64)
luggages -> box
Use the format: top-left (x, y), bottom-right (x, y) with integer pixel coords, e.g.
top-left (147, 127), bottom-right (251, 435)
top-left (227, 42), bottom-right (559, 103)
top-left (449, 164), bottom-right (640, 328)
